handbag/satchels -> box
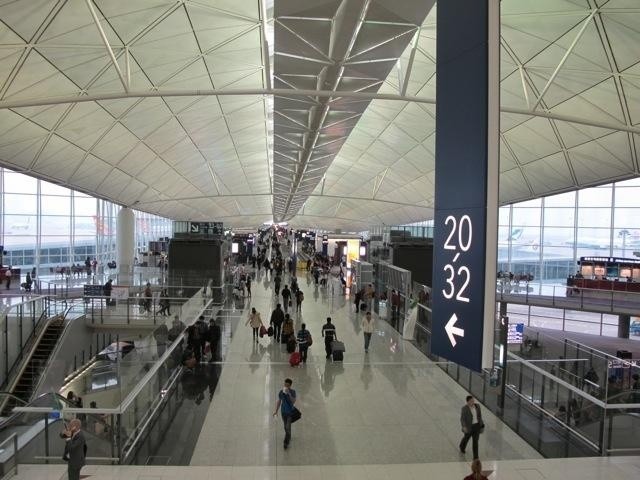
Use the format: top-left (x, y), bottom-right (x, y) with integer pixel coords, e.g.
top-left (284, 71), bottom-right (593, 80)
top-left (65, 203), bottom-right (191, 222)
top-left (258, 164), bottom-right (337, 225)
top-left (307, 336), bottom-right (312, 346)
top-left (331, 341), bottom-right (345, 353)
top-left (292, 408), bottom-right (301, 424)
top-left (260, 327), bottom-right (274, 338)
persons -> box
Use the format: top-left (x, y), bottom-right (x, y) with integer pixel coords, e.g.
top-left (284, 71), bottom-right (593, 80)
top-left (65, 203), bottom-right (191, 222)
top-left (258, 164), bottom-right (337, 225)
top-left (153, 315), bottom-right (187, 365)
top-left (273, 378), bottom-right (297, 451)
top-left (60, 419), bottom-right (86, 478)
top-left (336, 267), bottom-right (346, 286)
top-left (65, 391), bottom-right (100, 433)
top-left (104, 279), bottom-right (113, 304)
top-left (85, 256), bottom-right (97, 276)
top-left (156, 288), bottom-right (171, 316)
top-left (232, 271), bottom-right (337, 366)
top-left (353, 284), bottom-right (398, 314)
top-left (4, 267), bottom-right (12, 290)
top-left (576, 270), bottom-right (582, 278)
top-left (362, 311), bottom-right (373, 352)
top-left (459, 395), bottom-right (485, 459)
top-left (239, 231), bottom-right (293, 275)
top-left (144, 282), bottom-right (152, 311)
top-left (186, 315), bottom-right (220, 367)
top-left (25, 267), bottom-right (36, 292)
top-left (71, 263), bottom-right (84, 275)
top-left (626, 276), bottom-right (633, 282)
top-left (301, 235), bottom-right (333, 286)
top-left (464, 460), bottom-right (489, 480)
top-left (550, 356), bottom-right (640, 429)
top-left (496, 270), bottom-right (519, 284)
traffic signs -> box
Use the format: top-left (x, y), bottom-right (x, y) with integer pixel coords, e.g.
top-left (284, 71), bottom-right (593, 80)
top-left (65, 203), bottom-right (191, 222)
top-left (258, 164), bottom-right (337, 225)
top-left (190, 222), bottom-right (223, 235)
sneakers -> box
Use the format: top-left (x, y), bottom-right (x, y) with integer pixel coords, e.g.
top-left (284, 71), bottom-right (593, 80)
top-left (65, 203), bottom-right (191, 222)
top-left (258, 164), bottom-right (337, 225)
top-left (459, 445), bottom-right (466, 452)
top-left (284, 441), bottom-right (289, 449)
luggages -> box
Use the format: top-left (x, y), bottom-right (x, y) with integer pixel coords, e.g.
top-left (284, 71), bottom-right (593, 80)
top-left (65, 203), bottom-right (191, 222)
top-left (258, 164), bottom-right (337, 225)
top-left (334, 340), bottom-right (344, 361)
top-left (287, 340), bottom-right (295, 353)
top-left (289, 353), bottom-right (300, 366)
